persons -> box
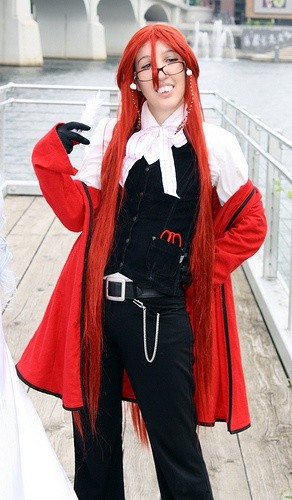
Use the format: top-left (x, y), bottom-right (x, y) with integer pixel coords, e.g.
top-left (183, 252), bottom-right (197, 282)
top-left (11, 20), bottom-right (268, 500)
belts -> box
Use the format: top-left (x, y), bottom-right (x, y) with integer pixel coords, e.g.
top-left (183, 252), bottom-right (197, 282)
top-left (103, 278), bottom-right (166, 302)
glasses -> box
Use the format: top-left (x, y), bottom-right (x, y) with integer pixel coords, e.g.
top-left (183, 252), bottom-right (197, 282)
top-left (132, 60), bottom-right (187, 81)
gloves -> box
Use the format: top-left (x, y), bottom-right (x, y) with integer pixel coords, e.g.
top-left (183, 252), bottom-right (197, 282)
top-left (56, 122), bottom-right (91, 154)
